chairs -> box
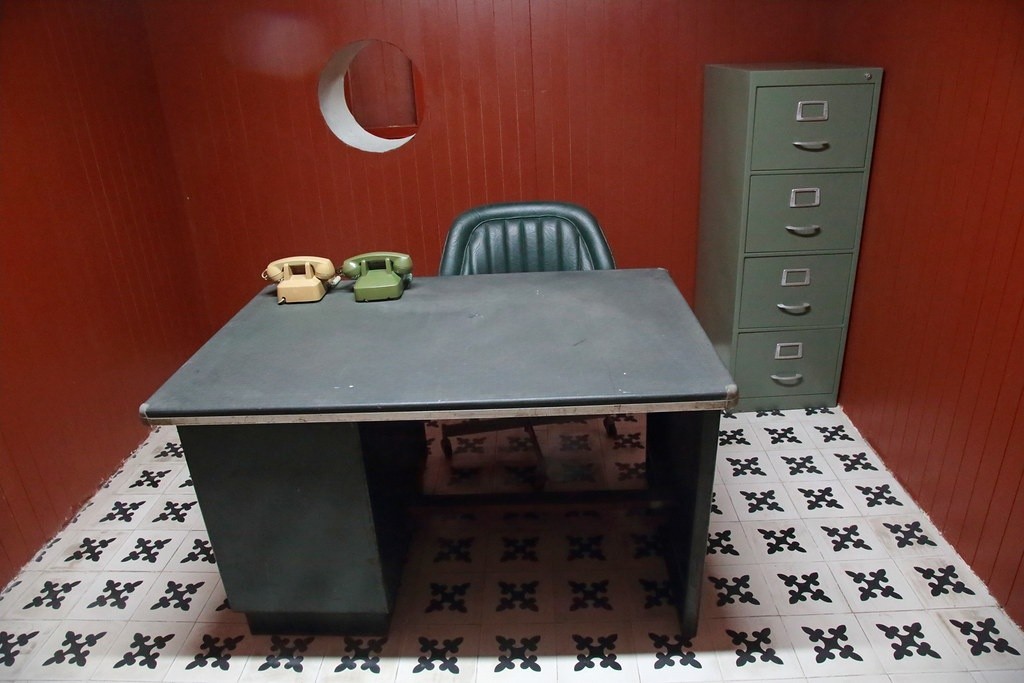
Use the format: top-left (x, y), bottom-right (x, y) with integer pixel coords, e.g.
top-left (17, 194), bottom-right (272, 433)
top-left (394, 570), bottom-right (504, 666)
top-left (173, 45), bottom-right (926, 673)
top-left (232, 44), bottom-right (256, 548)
top-left (436, 200), bottom-right (620, 460)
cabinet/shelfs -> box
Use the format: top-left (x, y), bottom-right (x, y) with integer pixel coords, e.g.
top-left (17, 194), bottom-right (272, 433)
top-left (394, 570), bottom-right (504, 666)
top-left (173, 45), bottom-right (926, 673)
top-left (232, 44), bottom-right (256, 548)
top-left (696, 63), bottom-right (887, 414)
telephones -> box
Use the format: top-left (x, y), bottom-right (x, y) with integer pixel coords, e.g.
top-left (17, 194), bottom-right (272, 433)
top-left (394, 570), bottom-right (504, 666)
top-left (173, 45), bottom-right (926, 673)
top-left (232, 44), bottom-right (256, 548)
top-left (261, 255), bottom-right (337, 305)
top-left (337, 251), bottom-right (414, 303)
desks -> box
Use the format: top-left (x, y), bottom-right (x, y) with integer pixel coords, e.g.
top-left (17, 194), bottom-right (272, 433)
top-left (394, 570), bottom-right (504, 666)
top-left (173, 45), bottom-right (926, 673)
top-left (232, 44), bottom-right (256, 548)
top-left (137, 266), bottom-right (741, 640)
